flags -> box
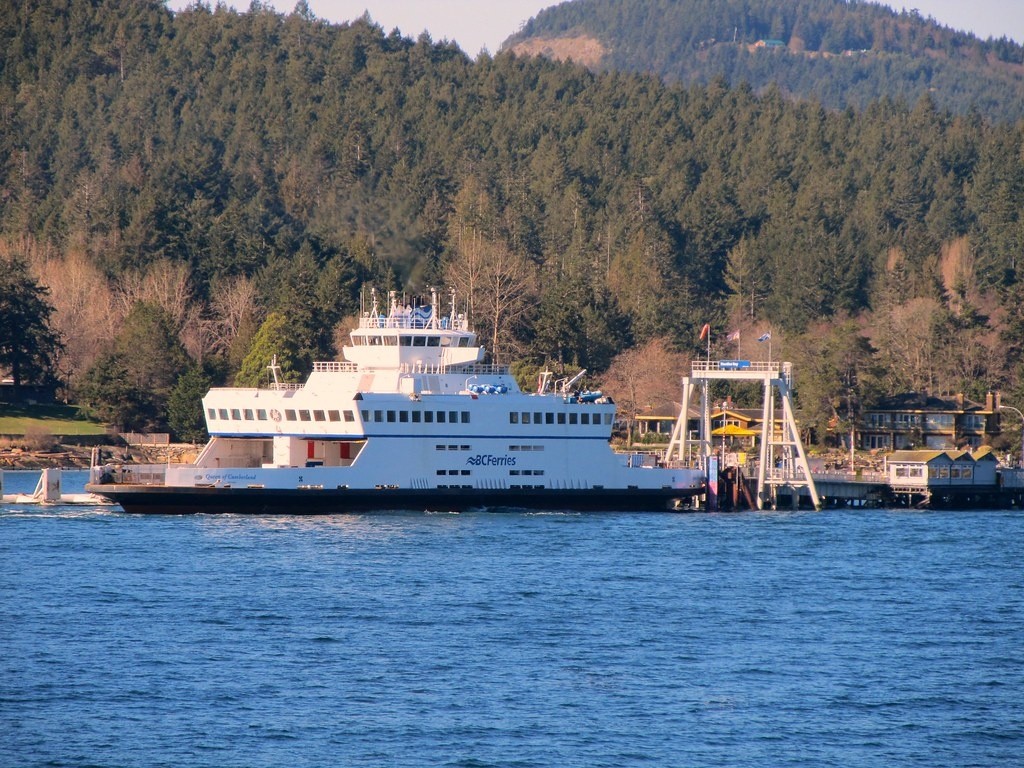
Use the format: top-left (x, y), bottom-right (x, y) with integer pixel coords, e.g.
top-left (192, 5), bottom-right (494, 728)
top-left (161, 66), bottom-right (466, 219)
top-left (757, 331), bottom-right (771, 341)
top-left (700, 323), bottom-right (708, 340)
top-left (727, 329), bottom-right (739, 341)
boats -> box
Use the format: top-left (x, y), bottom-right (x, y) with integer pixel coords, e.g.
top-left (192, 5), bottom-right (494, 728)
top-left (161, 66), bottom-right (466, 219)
top-left (84, 281), bottom-right (707, 514)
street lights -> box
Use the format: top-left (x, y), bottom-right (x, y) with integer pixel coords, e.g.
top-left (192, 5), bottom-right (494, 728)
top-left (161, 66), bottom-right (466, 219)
top-left (721, 401), bottom-right (729, 472)
top-left (998, 406), bottom-right (1023, 421)
